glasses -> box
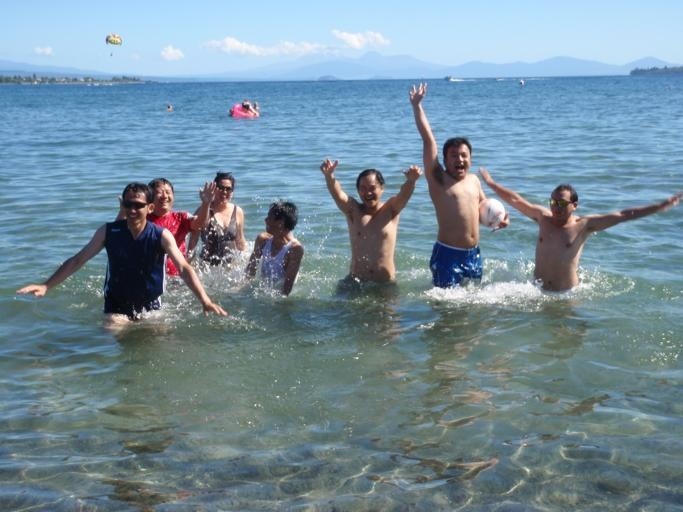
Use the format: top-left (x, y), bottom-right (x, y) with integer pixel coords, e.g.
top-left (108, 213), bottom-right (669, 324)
top-left (121, 200), bottom-right (150, 209)
top-left (549, 198), bottom-right (574, 208)
top-left (217, 183), bottom-right (233, 193)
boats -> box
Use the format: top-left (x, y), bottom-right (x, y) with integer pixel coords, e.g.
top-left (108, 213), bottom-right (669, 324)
top-left (229, 108), bottom-right (259, 119)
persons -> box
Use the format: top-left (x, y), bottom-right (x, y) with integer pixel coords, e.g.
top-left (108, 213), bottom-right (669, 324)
top-left (115, 175), bottom-right (221, 278)
top-left (402, 79), bottom-right (513, 291)
top-left (242, 198), bottom-right (307, 300)
top-left (12, 179), bottom-right (229, 332)
top-left (476, 163), bottom-right (683, 296)
top-left (316, 156), bottom-right (423, 296)
top-left (184, 169), bottom-right (249, 269)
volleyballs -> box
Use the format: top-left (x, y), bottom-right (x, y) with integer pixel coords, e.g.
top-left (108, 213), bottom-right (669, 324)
top-left (480, 199), bottom-right (505, 227)
